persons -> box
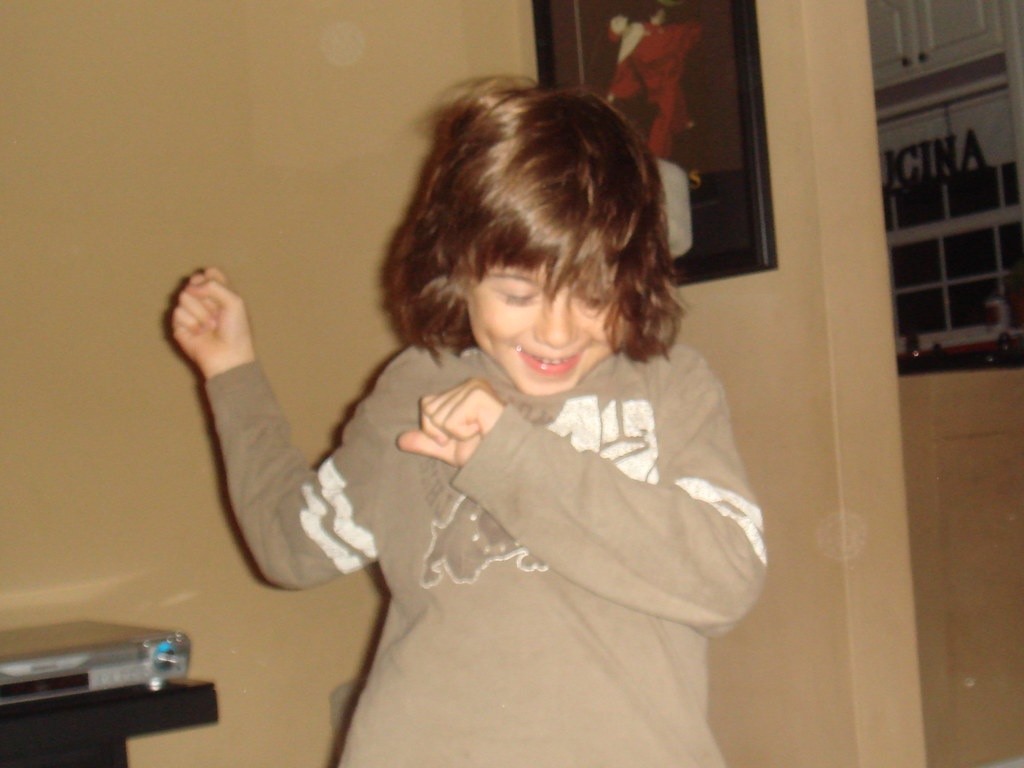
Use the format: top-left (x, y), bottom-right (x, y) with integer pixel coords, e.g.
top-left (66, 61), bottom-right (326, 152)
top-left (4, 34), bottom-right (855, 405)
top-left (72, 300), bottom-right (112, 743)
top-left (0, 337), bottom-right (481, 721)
top-left (605, 0), bottom-right (704, 161)
top-left (171, 90), bottom-right (769, 768)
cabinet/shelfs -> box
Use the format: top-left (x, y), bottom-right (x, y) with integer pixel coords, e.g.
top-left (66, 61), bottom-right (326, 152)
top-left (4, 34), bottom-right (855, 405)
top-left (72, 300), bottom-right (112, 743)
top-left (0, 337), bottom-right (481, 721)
top-left (865, 0), bottom-right (1024, 95)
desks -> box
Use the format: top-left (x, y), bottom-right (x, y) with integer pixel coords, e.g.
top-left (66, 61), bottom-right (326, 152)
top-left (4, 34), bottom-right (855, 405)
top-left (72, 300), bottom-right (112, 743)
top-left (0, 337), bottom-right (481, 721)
top-left (0, 681), bottom-right (222, 768)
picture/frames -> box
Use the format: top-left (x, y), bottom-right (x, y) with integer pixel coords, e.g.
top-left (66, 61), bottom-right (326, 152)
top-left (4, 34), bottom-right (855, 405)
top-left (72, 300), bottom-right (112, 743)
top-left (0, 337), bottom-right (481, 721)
top-left (530, 0), bottom-right (776, 284)
